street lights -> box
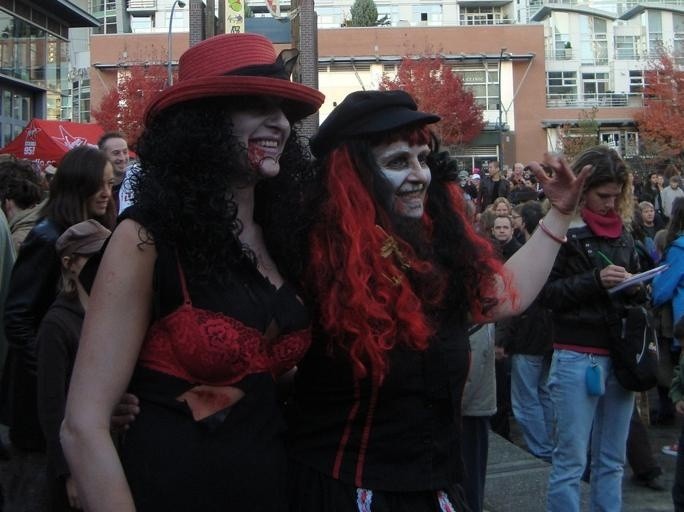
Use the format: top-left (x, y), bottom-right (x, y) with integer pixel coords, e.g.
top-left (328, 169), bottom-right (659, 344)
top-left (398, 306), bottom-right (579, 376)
top-left (165, 1), bottom-right (186, 87)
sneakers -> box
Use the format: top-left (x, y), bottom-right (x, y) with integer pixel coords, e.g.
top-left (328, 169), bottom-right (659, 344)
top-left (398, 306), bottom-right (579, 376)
top-left (661, 444), bottom-right (678, 456)
top-left (631, 468), bottom-right (676, 491)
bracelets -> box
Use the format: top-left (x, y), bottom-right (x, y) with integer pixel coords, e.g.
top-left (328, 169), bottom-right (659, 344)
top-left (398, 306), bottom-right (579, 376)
top-left (538, 217), bottom-right (567, 246)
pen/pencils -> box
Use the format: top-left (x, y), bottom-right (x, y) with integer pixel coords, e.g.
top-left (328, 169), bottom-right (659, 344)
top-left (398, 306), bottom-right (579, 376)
top-left (597, 251), bottom-right (614, 265)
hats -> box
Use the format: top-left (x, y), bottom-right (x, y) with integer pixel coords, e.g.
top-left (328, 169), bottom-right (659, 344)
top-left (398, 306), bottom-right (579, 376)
top-left (309, 91), bottom-right (441, 161)
top-left (142, 33), bottom-right (326, 129)
top-left (471, 174), bottom-right (480, 181)
top-left (56, 219), bottom-right (113, 258)
top-left (460, 171), bottom-right (469, 177)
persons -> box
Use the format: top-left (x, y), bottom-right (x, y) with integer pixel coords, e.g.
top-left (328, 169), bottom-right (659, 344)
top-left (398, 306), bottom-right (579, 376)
top-left (60, 31), bottom-right (325, 512)
top-left (6, 148), bottom-right (118, 450)
top-left (625, 154), bottom-right (684, 512)
top-left (538, 149), bottom-right (644, 511)
top-left (36, 219), bottom-right (110, 512)
top-left (462, 153), bottom-right (569, 509)
top-left (110, 94), bottom-right (592, 512)
top-left (0, 131), bottom-right (140, 316)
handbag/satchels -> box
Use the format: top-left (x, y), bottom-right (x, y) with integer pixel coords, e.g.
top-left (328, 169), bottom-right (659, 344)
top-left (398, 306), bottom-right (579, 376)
top-left (616, 300), bottom-right (661, 392)
